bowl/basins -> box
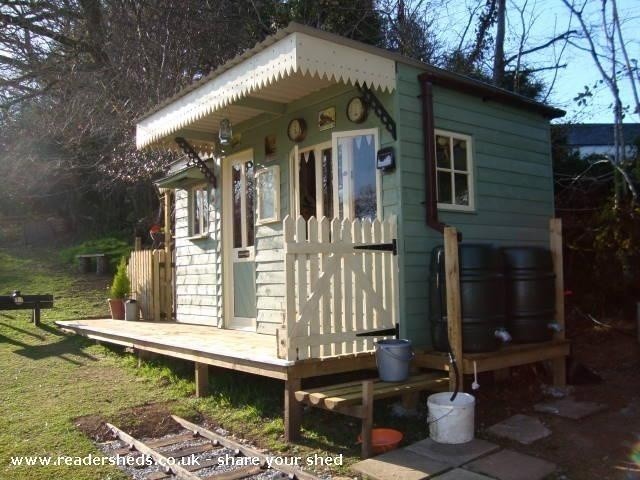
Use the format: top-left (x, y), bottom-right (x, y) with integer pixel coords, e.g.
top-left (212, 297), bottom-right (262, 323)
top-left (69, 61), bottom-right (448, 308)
top-left (357, 428), bottom-right (403, 453)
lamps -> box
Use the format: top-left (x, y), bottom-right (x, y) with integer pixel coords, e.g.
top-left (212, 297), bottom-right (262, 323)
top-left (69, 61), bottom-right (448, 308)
top-left (218, 107), bottom-right (233, 146)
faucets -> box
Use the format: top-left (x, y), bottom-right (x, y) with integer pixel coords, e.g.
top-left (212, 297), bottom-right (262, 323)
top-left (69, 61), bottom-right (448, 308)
top-left (494, 325), bottom-right (512, 345)
top-left (548, 319), bottom-right (564, 334)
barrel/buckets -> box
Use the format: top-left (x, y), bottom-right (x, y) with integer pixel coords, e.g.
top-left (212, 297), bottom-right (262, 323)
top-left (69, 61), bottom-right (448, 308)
top-left (373, 340), bottom-right (416, 383)
top-left (426, 392), bottom-right (477, 444)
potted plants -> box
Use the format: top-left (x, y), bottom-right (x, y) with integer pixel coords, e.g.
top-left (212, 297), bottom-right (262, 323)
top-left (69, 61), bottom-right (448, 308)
top-left (107, 256), bottom-right (131, 319)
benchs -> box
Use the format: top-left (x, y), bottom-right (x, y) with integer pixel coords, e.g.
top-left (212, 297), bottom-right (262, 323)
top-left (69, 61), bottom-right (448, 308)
top-left (0, 294), bottom-right (53, 324)
top-left (76, 253), bottom-right (110, 274)
